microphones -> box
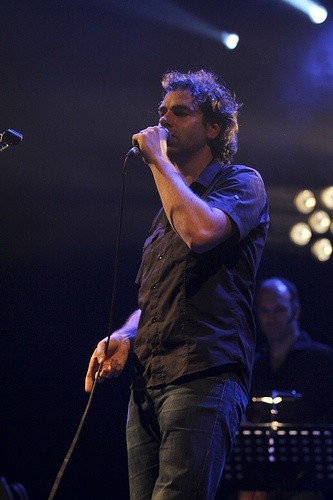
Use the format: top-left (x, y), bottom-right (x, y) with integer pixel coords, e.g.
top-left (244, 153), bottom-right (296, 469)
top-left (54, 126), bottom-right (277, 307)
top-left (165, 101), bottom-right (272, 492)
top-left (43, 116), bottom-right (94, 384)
top-left (127, 126), bottom-right (170, 158)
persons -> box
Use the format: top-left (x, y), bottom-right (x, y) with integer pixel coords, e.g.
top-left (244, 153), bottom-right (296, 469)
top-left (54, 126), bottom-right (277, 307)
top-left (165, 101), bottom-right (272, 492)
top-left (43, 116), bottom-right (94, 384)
top-left (233, 277), bottom-right (333, 499)
top-left (84, 69), bottom-right (269, 500)
